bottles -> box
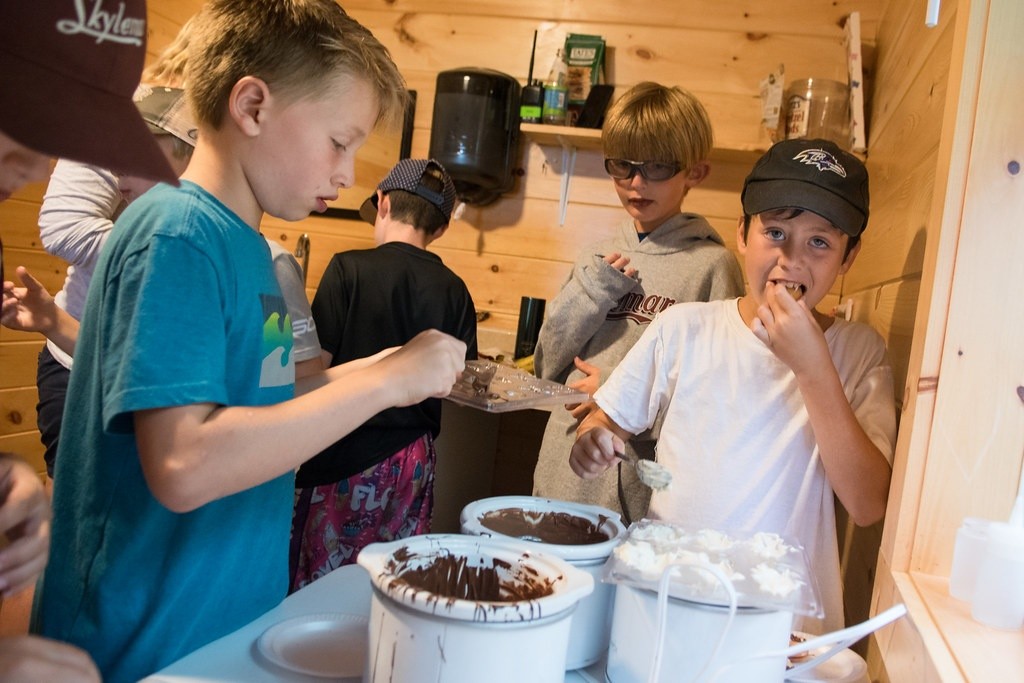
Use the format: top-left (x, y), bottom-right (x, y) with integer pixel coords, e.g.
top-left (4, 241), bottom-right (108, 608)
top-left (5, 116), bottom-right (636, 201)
top-left (542, 48), bottom-right (570, 126)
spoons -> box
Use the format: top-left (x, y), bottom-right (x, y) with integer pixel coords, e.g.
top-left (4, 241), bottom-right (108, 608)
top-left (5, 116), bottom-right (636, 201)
top-left (463, 366), bottom-right (500, 385)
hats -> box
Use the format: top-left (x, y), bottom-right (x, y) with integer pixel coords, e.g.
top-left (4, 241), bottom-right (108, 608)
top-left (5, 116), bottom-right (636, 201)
top-left (357, 157), bottom-right (457, 224)
top-left (0, 0), bottom-right (181, 190)
top-left (742, 136), bottom-right (871, 238)
top-left (130, 83), bottom-right (198, 148)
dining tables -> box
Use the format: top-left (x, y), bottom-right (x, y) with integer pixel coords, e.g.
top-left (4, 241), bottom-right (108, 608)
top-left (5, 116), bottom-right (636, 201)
top-left (130, 563), bottom-right (871, 683)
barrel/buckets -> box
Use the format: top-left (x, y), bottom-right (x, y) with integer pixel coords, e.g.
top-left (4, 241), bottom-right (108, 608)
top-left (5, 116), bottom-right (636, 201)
top-left (356, 532), bottom-right (594, 683)
top-left (604, 586), bottom-right (795, 683)
top-left (460, 497), bottom-right (627, 672)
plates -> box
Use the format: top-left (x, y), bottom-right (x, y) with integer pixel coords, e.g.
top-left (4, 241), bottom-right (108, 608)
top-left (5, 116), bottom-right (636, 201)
top-left (786, 630), bottom-right (867, 683)
top-left (257, 613), bottom-right (372, 678)
top-left (446, 360), bottom-right (589, 414)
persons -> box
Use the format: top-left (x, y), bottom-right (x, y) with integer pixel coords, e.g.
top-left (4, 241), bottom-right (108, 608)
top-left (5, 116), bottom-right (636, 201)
top-left (0, 86), bottom-right (323, 380)
top-left (0, 0), bottom-right (181, 683)
top-left (34, 11), bottom-right (203, 502)
top-left (568, 138), bottom-right (896, 643)
top-left (532, 81), bottom-right (747, 530)
top-left (286, 160), bottom-right (479, 598)
top-left (36, 0), bottom-right (466, 683)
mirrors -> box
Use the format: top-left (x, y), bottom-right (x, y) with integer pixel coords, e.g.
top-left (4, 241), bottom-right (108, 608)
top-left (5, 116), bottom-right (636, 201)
top-left (308, 89), bottom-right (417, 221)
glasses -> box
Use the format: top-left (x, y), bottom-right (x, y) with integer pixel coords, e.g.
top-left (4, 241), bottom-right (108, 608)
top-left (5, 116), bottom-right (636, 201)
top-left (602, 156), bottom-right (691, 183)
